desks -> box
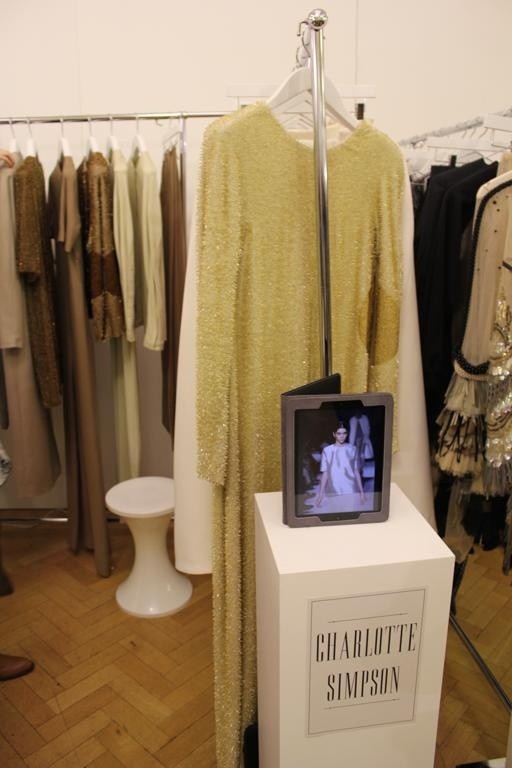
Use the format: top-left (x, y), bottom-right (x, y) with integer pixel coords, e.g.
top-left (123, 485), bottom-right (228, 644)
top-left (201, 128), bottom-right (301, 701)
top-left (254, 486), bottom-right (458, 766)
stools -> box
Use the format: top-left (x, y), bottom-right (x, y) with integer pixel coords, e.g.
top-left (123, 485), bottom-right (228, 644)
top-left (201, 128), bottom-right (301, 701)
top-left (106, 474), bottom-right (195, 618)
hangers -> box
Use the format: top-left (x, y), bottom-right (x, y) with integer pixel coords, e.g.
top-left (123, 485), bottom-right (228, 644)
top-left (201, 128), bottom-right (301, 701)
top-left (397, 104), bottom-right (512, 194)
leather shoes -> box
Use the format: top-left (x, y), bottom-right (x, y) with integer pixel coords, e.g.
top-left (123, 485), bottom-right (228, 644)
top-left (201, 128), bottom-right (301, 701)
top-left (0, 654), bottom-right (34, 680)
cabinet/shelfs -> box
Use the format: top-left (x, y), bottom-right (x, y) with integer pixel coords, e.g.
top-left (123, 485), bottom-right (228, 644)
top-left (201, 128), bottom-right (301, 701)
top-left (0, 114), bottom-right (184, 160)
top-left (265, 19), bottom-right (359, 143)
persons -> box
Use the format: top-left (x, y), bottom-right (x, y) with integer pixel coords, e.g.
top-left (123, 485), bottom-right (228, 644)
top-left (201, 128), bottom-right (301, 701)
top-left (0, 651), bottom-right (35, 686)
top-left (311, 417), bottom-right (368, 508)
